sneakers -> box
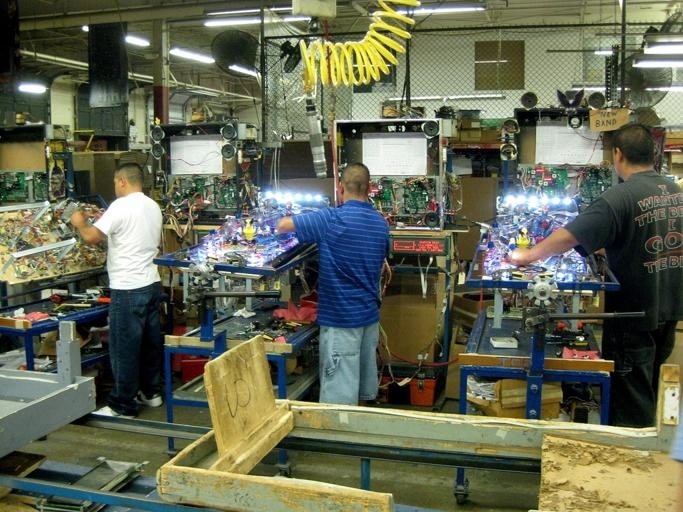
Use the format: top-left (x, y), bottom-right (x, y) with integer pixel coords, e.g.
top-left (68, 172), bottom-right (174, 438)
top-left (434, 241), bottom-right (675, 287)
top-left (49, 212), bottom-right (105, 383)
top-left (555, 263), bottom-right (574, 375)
top-left (90, 405), bottom-right (136, 420)
top-left (130, 393), bottom-right (164, 407)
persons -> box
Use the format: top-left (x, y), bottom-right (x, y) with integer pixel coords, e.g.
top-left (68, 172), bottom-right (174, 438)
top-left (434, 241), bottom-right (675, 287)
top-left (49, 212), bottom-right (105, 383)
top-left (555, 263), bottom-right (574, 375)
top-left (277, 163), bottom-right (389, 405)
top-left (70, 162), bottom-right (162, 419)
top-left (508, 125), bottom-right (682, 426)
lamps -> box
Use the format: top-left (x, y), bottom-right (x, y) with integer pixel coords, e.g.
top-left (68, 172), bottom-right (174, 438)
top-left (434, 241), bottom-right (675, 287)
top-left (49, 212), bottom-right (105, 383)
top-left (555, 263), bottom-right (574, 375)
top-left (202, 1), bottom-right (491, 28)
top-left (593, 32), bottom-right (683, 100)
top-left (81, 23), bottom-right (260, 83)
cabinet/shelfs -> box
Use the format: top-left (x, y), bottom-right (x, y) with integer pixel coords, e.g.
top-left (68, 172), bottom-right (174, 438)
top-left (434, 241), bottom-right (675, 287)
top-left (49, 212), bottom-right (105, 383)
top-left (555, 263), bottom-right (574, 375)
top-left (152, 235), bottom-right (324, 478)
top-left (457, 235), bottom-right (621, 503)
top-left (3, 233), bottom-right (176, 442)
